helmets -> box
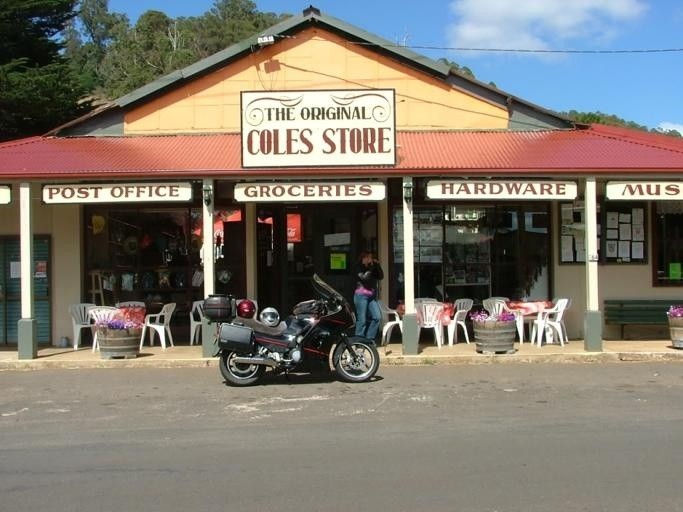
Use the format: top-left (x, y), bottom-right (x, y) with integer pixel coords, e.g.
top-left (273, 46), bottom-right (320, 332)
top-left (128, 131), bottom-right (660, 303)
top-left (259, 307), bottom-right (280, 326)
top-left (238, 300), bottom-right (256, 317)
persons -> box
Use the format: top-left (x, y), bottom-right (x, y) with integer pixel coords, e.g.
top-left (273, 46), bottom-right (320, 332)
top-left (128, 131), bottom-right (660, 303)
top-left (354, 251), bottom-right (384, 338)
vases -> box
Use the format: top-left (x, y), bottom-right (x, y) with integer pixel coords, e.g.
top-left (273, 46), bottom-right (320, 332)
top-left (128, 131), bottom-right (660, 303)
top-left (667, 314), bottom-right (682, 349)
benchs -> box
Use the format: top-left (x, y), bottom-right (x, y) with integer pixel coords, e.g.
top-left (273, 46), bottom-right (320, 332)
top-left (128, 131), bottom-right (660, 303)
top-left (603, 299), bottom-right (682, 340)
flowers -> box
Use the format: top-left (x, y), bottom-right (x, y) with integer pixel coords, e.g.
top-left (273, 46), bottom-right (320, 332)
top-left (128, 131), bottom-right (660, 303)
top-left (664, 302), bottom-right (683, 318)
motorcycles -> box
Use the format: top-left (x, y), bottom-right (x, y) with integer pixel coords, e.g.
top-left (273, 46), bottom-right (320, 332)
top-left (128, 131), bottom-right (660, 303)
top-left (199, 272), bottom-right (379, 387)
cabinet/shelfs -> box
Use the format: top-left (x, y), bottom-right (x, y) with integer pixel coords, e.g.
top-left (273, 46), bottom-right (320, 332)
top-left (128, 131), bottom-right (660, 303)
top-left (440, 221), bottom-right (492, 302)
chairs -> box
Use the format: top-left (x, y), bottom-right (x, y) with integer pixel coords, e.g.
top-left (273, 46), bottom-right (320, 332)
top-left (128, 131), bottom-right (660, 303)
top-left (190, 301), bottom-right (206, 346)
top-left (140, 302), bottom-right (177, 352)
top-left (235, 300), bottom-right (258, 320)
top-left (377, 299), bottom-right (403, 346)
top-left (482, 297), bottom-right (569, 350)
top-left (413, 298), bottom-right (445, 348)
top-left (115, 301), bottom-right (145, 308)
top-left (88, 306), bottom-right (124, 353)
top-left (68, 304), bottom-right (101, 351)
top-left (434, 299), bottom-right (473, 344)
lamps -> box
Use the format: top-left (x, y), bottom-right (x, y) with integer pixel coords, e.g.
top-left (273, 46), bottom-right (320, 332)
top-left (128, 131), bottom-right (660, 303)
top-left (200, 178), bottom-right (211, 206)
top-left (402, 178), bottom-right (414, 204)
